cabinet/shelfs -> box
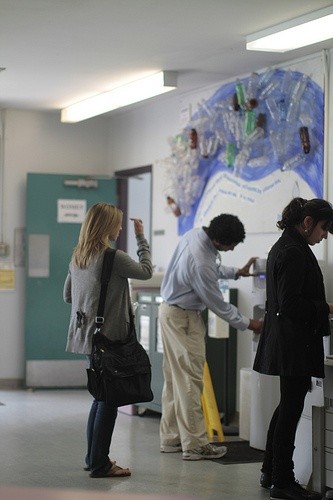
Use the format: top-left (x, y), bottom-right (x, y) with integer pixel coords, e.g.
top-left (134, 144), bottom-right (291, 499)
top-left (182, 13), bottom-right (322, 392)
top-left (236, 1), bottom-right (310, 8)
top-left (131, 280), bottom-right (238, 427)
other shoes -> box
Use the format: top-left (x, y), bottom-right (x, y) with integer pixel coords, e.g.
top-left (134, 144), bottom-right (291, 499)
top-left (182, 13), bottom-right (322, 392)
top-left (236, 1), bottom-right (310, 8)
top-left (89, 464), bottom-right (131, 478)
top-left (83, 457), bottom-right (116, 471)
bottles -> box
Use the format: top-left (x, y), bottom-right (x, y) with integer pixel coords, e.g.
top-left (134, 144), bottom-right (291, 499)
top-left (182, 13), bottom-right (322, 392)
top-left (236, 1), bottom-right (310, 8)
top-left (131, 302), bottom-right (140, 314)
top-left (253, 315), bottom-right (264, 342)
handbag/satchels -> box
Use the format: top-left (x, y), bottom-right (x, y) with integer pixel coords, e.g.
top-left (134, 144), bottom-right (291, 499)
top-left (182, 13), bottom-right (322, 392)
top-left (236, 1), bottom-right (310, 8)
top-left (86, 327), bottom-right (154, 408)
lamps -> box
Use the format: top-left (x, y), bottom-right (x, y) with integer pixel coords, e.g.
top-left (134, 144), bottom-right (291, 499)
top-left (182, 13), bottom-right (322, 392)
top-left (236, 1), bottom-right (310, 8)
top-left (61, 71), bottom-right (179, 125)
top-left (244, 4), bottom-right (333, 56)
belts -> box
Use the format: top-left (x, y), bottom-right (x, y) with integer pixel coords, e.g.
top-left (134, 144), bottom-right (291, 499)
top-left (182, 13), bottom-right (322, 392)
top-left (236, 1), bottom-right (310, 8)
top-left (170, 304), bottom-right (201, 311)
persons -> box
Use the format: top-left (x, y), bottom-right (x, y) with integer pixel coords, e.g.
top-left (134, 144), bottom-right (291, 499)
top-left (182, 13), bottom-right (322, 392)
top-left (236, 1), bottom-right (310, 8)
top-left (251, 197), bottom-right (333, 500)
top-left (62, 202), bottom-right (154, 479)
top-left (158, 213), bottom-right (264, 461)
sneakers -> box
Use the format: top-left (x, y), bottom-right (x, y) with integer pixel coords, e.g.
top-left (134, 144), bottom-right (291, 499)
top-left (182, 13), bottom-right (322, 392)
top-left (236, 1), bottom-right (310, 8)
top-left (160, 443), bottom-right (182, 452)
top-left (270, 480), bottom-right (323, 500)
top-left (260, 477), bottom-right (272, 488)
top-left (182, 444), bottom-right (227, 460)
